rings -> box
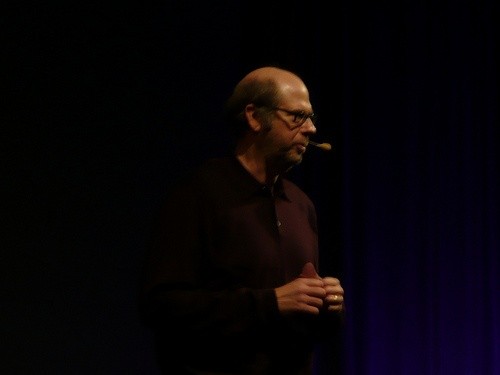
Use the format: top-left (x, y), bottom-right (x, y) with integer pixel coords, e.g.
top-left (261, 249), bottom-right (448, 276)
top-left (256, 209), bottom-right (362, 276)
top-left (334, 295), bottom-right (338, 304)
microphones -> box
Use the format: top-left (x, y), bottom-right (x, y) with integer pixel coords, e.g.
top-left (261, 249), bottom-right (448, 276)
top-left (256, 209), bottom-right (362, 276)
top-left (307, 141), bottom-right (331, 150)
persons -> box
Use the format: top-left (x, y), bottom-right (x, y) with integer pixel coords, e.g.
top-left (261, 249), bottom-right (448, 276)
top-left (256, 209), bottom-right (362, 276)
top-left (139, 67), bottom-right (345, 375)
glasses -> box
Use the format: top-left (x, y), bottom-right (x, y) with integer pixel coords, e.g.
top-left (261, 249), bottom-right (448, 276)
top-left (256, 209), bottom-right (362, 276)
top-left (263, 106), bottom-right (315, 123)
